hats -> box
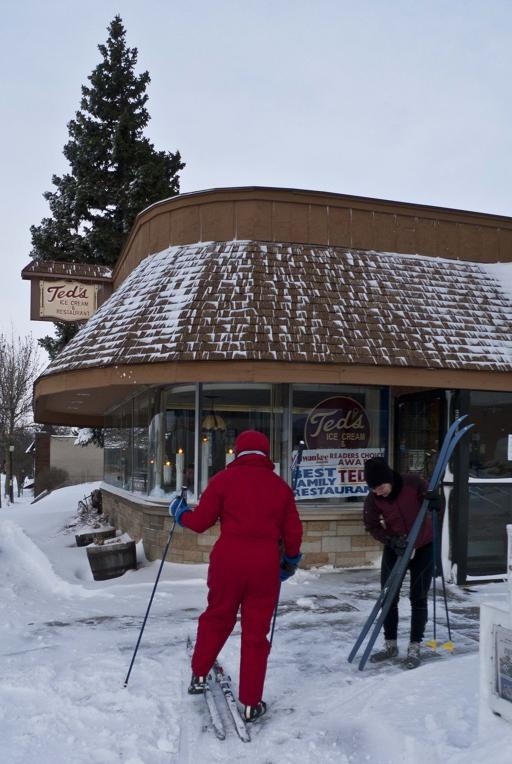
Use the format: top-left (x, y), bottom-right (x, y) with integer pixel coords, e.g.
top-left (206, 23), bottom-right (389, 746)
top-left (364, 457), bottom-right (393, 489)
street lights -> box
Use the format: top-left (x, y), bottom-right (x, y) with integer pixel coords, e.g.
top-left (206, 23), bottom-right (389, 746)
top-left (8, 440), bottom-right (15, 506)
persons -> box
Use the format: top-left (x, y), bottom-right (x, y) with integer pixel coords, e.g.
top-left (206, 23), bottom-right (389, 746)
top-left (167, 429), bottom-right (304, 724)
top-left (361, 455), bottom-right (444, 671)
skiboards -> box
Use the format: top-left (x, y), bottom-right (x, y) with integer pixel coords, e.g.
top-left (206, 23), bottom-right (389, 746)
top-left (186, 634), bottom-right (251, 743)
top-left (347, 414), bottom-right (475, 671)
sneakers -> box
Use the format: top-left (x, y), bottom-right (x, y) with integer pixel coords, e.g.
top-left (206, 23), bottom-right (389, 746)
top-left (407, 646), bottom-right (421, 668)
top-left (244, 701), bottom-right (266, 721)
top-left (370, 647), bottom-right (399, 663)
top-left (188, 675), bottom-right (207, 693)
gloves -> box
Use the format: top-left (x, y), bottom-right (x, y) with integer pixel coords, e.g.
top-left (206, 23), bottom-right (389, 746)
top-left (168, 496), bottom-right (192, 527)
top-left (391, 534), bottom-right (409, 557)
top-left (279, 553), bottom-right (304, 582)
top-left (422, 489), bottom-right (445, 511)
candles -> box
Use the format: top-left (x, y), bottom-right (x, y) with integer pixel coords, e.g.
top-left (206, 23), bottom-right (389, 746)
top-left (162, 447), bottom-right (184, 492)
top-left (224, 448), bottom-right (235, 467)
top-left (200, 437), bottom-right (208, 494)
